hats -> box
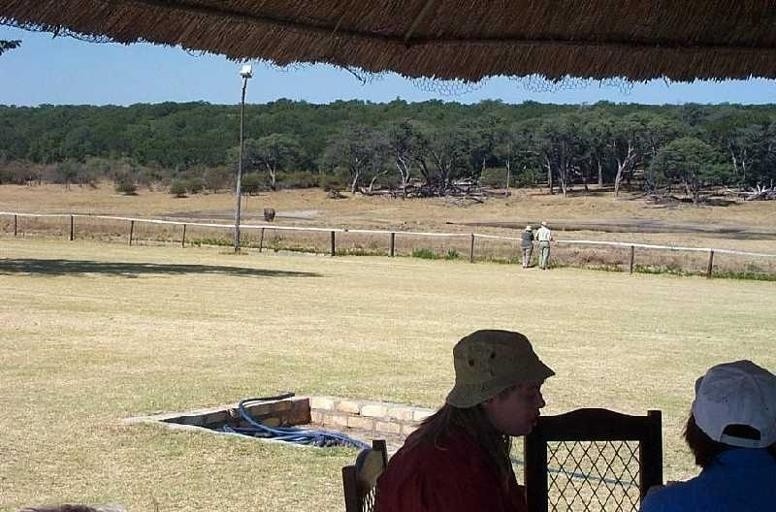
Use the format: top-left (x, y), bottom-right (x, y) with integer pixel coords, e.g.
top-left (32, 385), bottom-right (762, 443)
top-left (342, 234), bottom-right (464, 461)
top-left (524, 226), bottom-right (532, 232)
top-left (541, 222), bottom-right (547, 227)
top-left (691, 359), bottom-right (776, 449)
top-left (445, 329), bottom-right (556, 408)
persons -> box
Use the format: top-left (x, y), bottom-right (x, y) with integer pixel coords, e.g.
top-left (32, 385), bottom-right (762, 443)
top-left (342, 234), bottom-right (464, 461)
top-left (637, 358), bottom-right (776, 510)
top-left (534, 220), bottom-right (554, 270)
top-left (518, 224), bottom-right (535, 270)
top-left (372, 328), bottom-right (557, 510)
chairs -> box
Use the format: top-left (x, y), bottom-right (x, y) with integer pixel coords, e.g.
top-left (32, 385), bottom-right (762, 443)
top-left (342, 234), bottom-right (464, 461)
top-left (342, 439), bottom-right (388, 512)
top-left (524, 409), bottom-right (662, 512)
